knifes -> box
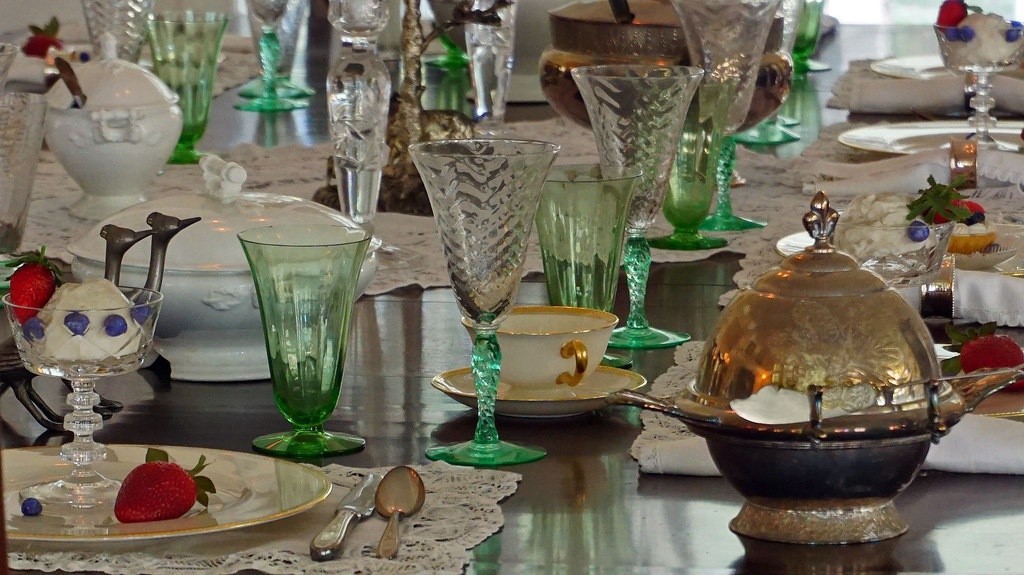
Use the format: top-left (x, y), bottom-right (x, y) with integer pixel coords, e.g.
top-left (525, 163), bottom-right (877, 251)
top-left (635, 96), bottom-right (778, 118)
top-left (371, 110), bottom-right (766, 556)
top-left (307, 476), bottom-right (385, 560)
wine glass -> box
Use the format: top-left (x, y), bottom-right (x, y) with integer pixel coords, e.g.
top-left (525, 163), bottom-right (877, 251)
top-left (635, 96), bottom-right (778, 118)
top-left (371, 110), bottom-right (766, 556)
top-left (82, 1), bottom-right (827, 464)
top-left (4, 282), bottom-right (165, 513)
top-left (933, 22), bottom-right (1023, 153)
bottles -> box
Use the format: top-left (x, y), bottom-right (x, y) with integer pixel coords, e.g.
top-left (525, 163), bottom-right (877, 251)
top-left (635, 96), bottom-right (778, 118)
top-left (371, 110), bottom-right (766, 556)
top-left (46, 32), bottom-right (191, 217)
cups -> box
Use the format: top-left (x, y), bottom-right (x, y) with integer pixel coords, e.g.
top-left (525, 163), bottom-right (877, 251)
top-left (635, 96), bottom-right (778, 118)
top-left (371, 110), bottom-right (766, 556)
top-left (535, 162), bottom-right (643, 316)
top-left (459, 304), bottom-right (620, 399)
top-left (831, 206), bottom-right (955, 287)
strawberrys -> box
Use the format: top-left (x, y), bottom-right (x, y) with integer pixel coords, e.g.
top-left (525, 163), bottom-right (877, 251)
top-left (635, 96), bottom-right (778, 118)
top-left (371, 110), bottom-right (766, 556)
top-left (937, 0), bottom-right (981, 33)
top-left (22, 17), bottom-right (64, 58)
top-left (114, 447), bottom-right (218, 523)
top-left (908, 176), bottom-right (986, 224)
top-left (941, 318), bottom-right (1024, 393)
top-left (4, 244), bottom-right (64, 326)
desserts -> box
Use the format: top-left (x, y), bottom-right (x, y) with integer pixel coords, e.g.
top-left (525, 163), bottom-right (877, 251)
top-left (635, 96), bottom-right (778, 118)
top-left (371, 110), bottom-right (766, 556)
top-left (948, 220), bottom-right (998, 254)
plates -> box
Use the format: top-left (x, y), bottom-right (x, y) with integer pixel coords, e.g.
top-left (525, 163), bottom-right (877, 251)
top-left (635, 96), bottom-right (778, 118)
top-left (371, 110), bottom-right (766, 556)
top-left (934, 343), bottom-right (1024, 417)
top-left (1, 445), bottom-right (334, 558)
top-left (431, 365), bottom-right (650, 418)
top-left (839, 119), bottom-right (1024, 156)
top-left (776, 224), bottom-right (1024, 278)
top-left (869, 53), bottom-right (1024, 77)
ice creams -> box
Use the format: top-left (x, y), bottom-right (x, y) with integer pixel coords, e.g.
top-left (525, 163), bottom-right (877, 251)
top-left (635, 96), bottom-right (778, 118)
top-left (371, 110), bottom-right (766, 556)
top-left (833, 192), bottom-right (940, 257)
top-left (24, 278), bottom-right (151, 363)
top-left (943, 12), bottom-right (1023, 67)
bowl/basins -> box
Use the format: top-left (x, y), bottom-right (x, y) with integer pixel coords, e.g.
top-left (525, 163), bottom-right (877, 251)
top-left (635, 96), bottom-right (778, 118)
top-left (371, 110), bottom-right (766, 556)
top-left (947, 229), bottom-right (997, 253)
top-left (64, 154), bottom-right (399, 382)
top-left (606, 189), bottom-right (1024, 546)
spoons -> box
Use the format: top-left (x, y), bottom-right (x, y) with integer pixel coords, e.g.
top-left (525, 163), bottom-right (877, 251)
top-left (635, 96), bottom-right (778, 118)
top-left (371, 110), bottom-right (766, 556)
top-left (374, 465), bottom-right (425, 559)
